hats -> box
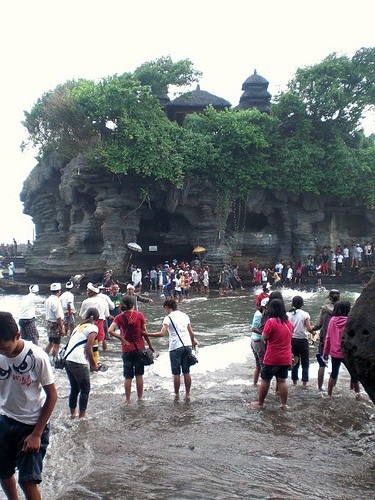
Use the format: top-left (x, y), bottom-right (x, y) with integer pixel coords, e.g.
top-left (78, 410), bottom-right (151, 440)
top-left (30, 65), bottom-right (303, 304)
top-left (261, 298), bottom-right (269, 306)
top-left (330, 289), bottom-right (340, 295)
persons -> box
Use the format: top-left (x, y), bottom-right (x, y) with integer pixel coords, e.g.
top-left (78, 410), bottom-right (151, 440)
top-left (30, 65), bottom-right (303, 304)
top-left (62, 308), bottom-right (99, 419)
top-left (251, 298), bottom-right (295, 408)
top-left (142, 299), bottom-right (196, 401)
top-left (0, 241), bottom-right (375, 398)
top-left (0, 311), bottom-right (58, 500)
top-left (107, 295), bottom-right (155, 405)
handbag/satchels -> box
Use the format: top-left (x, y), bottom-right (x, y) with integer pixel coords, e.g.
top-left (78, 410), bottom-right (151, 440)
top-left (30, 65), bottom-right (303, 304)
top-left (138, 349), bottom-right (154, 365)
top-left (188, 354), bottom-right (198, 365)
top-left (19, 320), bottom-right (29, 326)
top-left (54, 354), bottom-right (66, 369)
